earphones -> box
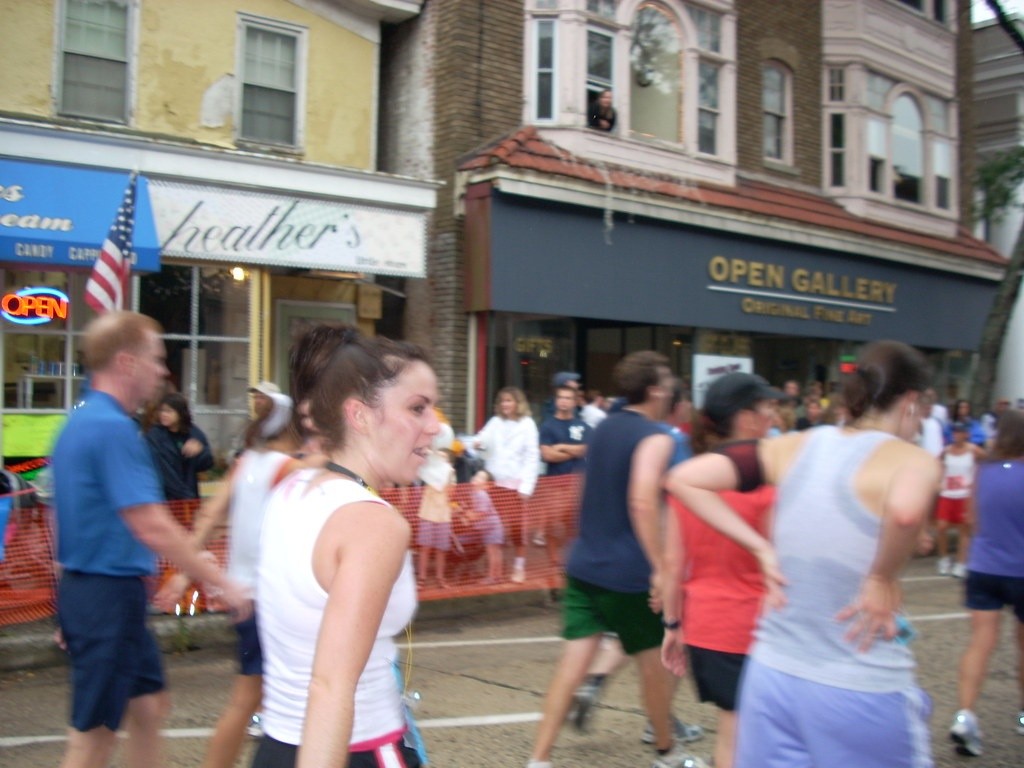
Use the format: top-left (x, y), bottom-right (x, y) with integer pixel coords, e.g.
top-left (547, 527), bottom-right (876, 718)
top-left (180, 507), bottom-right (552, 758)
top-left (910, 402), bottom-right (915, 414)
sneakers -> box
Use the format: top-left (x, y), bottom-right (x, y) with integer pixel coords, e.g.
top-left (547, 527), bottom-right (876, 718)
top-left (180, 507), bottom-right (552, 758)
top-left (569, 686), bottom-right (598, 736)
top-left (1011, 711), bottom-right (1024, 734)
top-left (642, 713), bottom-right (704, 743)
top-left (949, 710), bottom-right (986, 757)
top-left (645, 745), bottom-right (712, 768)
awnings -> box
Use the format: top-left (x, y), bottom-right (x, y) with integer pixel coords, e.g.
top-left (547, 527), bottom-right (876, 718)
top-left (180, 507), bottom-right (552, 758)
top-left (0, 159), bottom-right (161, 272)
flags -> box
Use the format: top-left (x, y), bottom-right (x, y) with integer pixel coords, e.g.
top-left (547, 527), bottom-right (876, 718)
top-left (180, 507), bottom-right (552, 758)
top-left (80, 174), bottom-right (138, 317)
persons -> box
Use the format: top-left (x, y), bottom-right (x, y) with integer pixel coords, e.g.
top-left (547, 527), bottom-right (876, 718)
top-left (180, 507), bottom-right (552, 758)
top-left (775, 375), bottom-right (1024, 580)
top-left (521, 340), bottom-right (938, 768)
top-left (137, 380), bottom-right (302, 768)
top-left (411, 369), bottom-right (627, 593)
top-left (949, 409), bottom-right (1024, 757)
top-left (248, 323), bottom-right (441, 768)
top-left (52, 312), bottom-right (253, 768)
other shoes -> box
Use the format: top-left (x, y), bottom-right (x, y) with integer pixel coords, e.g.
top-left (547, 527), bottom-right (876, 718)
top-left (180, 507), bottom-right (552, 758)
top-left (509, 569), bottom-right (526, 584)
top-left (532, 536), bottom-right (549, 547)
top-left (950, 563), bottom-right (967, 580)
top-left (933, 556), bottom-right (953, 575)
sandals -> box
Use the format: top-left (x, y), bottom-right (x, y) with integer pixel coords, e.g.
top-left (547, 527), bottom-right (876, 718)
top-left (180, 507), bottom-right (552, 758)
top-left (435, 579), bottom-right (450, 590)
top-left (476, 575), bottom-right (504, 586)
top-left (414, 577), bottom-right (429, 591)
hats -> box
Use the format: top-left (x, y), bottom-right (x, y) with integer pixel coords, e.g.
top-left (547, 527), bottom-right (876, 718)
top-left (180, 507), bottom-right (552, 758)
top-left (698, 371), bottom-right (793, 413)
top-left (550, 372), bottom-right (582, 387)
top-left (245, 382), bottom-right (282, 396)
top-left (948, 420), bottom-right (970, 432)
